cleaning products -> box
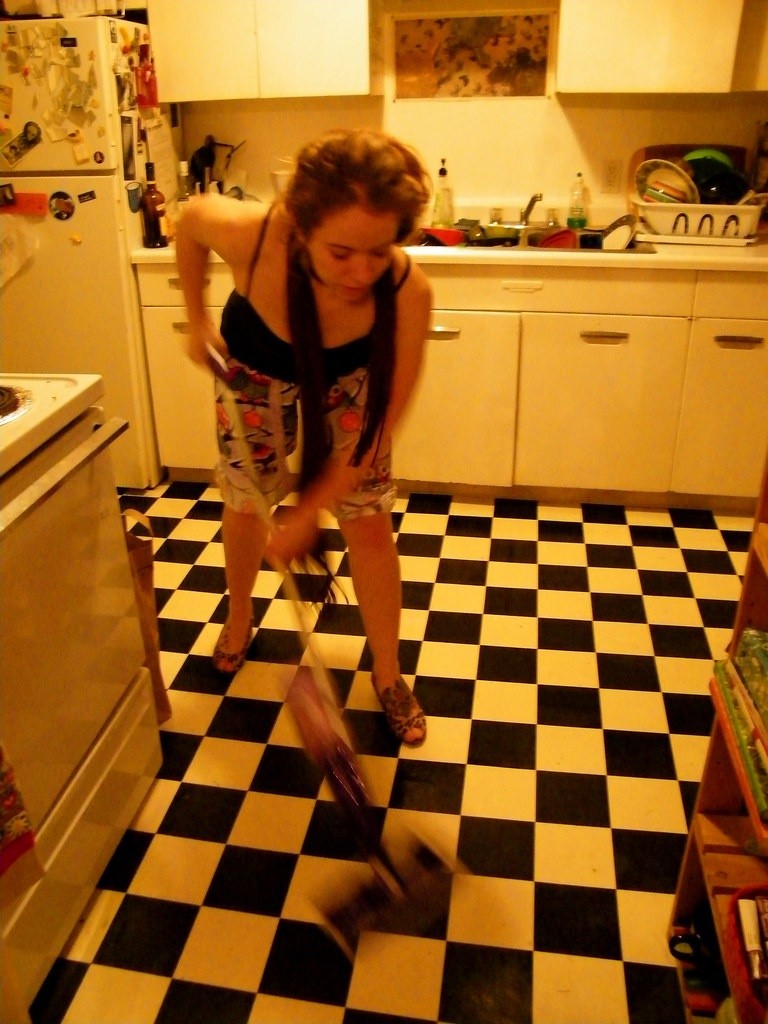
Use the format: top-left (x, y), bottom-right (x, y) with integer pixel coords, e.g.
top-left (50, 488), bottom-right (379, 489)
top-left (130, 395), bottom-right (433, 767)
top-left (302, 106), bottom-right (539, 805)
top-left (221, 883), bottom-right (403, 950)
top-left (567, 173), bottom-right (588, 229)
top-left (432, 158), bottom-right (455, 231)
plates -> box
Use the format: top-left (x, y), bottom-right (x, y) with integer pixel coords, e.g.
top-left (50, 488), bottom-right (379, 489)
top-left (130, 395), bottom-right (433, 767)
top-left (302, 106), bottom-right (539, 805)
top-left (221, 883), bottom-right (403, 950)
top-left (601, 215), bottom-right (638, 249)
top-left (634, 161), bottom-right (699, 204)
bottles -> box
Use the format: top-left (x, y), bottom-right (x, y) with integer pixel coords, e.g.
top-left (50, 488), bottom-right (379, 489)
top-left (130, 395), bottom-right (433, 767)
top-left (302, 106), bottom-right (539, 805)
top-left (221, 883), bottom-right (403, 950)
top-left (177, 160), bottom-right (198, 214)
top-left (140, 162), bottom-right (168, 248)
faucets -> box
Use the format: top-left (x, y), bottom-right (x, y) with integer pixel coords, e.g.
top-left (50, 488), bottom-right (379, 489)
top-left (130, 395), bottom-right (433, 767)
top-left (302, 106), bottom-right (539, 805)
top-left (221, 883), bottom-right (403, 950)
top-left (520, 194), bottom-right (542, 226)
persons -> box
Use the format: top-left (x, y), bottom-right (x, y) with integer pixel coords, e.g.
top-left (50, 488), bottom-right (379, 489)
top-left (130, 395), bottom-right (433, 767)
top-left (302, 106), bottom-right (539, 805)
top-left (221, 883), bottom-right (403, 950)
top-left (174, 125), bottom-right (433, 747)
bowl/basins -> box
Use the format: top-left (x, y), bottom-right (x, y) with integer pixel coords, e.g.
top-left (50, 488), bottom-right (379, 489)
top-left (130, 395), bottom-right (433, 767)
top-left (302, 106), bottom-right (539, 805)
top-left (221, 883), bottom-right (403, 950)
top-left (683, 148), bottom-right (734, 181)
top-left (701, 174), bottom-right (749, 207)
top-left (484, 227), bottom-right (519, 238)
top-left (420, 228), bottom-right (465, 245)
top-left (468, 226), bottom-right (485, 243)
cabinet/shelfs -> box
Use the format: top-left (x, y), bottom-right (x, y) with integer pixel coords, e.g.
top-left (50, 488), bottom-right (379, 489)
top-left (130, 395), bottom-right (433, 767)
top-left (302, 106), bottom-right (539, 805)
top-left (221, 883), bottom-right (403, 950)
top-left (553, 0), bottom-right (767, 96)
top-left (146, 2), bottom-right (383, 104)
top-left (131, 261), bottom-right (768, 518)
top-left (661, 441), bottom-right (768, 1024)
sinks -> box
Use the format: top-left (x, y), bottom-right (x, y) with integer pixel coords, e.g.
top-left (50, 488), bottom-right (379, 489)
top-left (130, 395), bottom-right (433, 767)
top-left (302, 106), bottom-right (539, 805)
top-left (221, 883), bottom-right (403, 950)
top-left (526, 226), bottom-right (658, 255)
top-left (407, 224), bottom-right (523, 250)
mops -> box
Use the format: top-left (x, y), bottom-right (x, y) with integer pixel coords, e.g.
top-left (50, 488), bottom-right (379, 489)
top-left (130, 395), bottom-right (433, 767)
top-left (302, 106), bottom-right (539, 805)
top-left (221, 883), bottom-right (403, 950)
top-left (207, 342), bottom-right (468, 963)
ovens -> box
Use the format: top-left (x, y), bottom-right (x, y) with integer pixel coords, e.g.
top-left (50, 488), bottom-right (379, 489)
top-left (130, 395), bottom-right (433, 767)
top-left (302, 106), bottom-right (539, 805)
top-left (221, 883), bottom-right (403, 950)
top-left (0, 407), bottom-right (161, 1013)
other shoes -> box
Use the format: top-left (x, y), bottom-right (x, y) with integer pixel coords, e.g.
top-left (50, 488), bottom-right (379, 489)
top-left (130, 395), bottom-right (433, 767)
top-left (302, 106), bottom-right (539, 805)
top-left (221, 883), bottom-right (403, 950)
top-left (214, 610), bottom-right (255, 674)
top-left (371, 672), bottom-right (427, 745)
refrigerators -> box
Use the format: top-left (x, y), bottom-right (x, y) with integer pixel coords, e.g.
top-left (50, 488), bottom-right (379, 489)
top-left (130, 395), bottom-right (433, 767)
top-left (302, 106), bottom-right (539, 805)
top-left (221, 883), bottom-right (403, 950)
top-left (0, 16), bottom-right (183, 489)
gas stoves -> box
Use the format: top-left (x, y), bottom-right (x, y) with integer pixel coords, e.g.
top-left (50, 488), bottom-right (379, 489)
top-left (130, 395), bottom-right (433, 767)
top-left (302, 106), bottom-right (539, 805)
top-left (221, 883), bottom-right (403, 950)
top-left (0, 373), bottom-right (105, 477)
